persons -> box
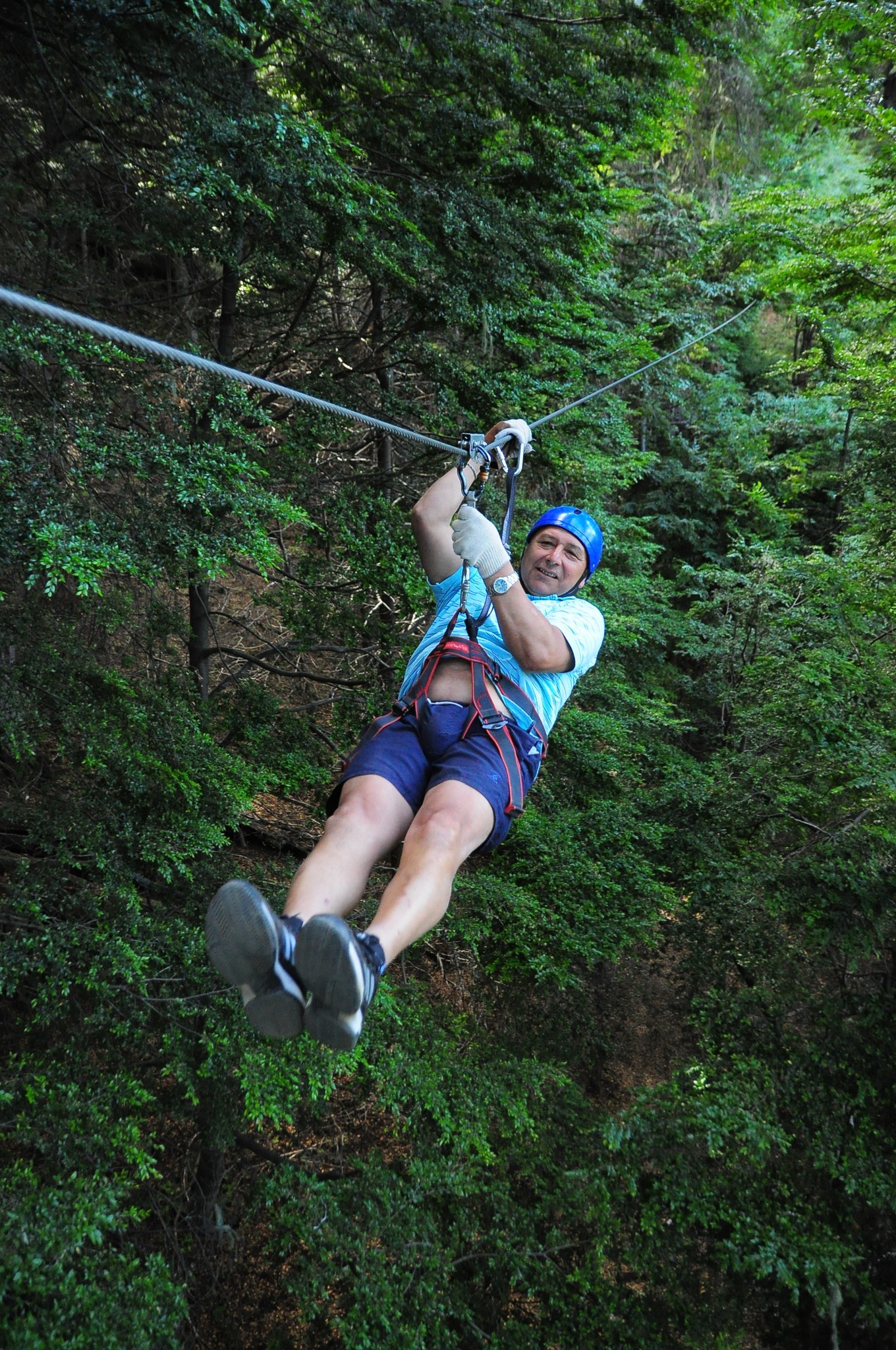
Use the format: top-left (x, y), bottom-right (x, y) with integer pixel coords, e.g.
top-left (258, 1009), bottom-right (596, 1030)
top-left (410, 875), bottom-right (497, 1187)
top-left (205, 416), bottom-right (604, 1052)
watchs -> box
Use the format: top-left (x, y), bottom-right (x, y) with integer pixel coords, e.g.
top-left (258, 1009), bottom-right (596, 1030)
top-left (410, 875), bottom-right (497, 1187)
top-left (486, 571), bottom-right (520, 597)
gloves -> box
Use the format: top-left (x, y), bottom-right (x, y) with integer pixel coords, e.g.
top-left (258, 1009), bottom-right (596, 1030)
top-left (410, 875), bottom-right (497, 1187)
top-left (451, 503), bottom-right (511, 579)
top-left (483, 419), bottom-right (533, 462)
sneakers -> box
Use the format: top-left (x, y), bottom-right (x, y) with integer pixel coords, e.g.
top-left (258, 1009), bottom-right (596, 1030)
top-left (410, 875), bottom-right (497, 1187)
top-left (204, 879), bottom-right (307, 1038)
top-left (295, 912), bottom-right (386, 1052)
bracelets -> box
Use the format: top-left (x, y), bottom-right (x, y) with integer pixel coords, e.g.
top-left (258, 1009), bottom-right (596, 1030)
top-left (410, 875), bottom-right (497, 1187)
top-left (468, 463), bottom-right (477, 480)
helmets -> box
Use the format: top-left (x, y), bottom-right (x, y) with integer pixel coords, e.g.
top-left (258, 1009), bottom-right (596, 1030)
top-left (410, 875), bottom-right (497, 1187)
top-left (525, 506), bottom-right (603, 579)
